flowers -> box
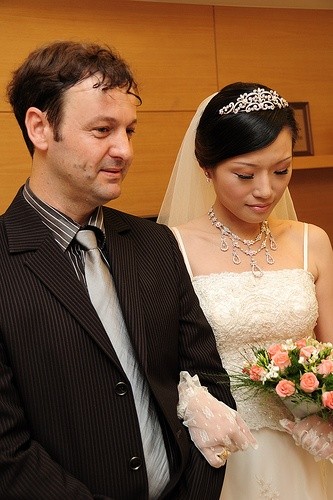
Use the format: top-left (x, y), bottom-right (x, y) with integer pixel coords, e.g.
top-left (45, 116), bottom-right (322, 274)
top-left (210, 335), bottom-right (333, 427)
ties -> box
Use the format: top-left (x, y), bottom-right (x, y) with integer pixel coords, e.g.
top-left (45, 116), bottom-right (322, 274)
top-left (75, 230), bottom-right (172, 500)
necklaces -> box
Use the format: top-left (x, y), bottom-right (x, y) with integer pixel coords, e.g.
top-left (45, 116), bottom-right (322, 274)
top-left (208, 206), bottom-right (279, 277)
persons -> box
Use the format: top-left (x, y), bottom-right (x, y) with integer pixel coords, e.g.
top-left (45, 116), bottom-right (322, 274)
top-left (0, 42), bottom-right (237, 500)
top-left (157, 81), bottom-right (332, 500)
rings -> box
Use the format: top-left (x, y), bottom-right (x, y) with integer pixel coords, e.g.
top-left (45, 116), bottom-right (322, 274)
top-left (221, 451), bottom-right (229, 460)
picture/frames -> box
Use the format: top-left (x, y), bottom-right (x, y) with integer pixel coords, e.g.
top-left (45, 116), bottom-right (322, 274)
top-left (287, 102), bottom-right (315, 157)
